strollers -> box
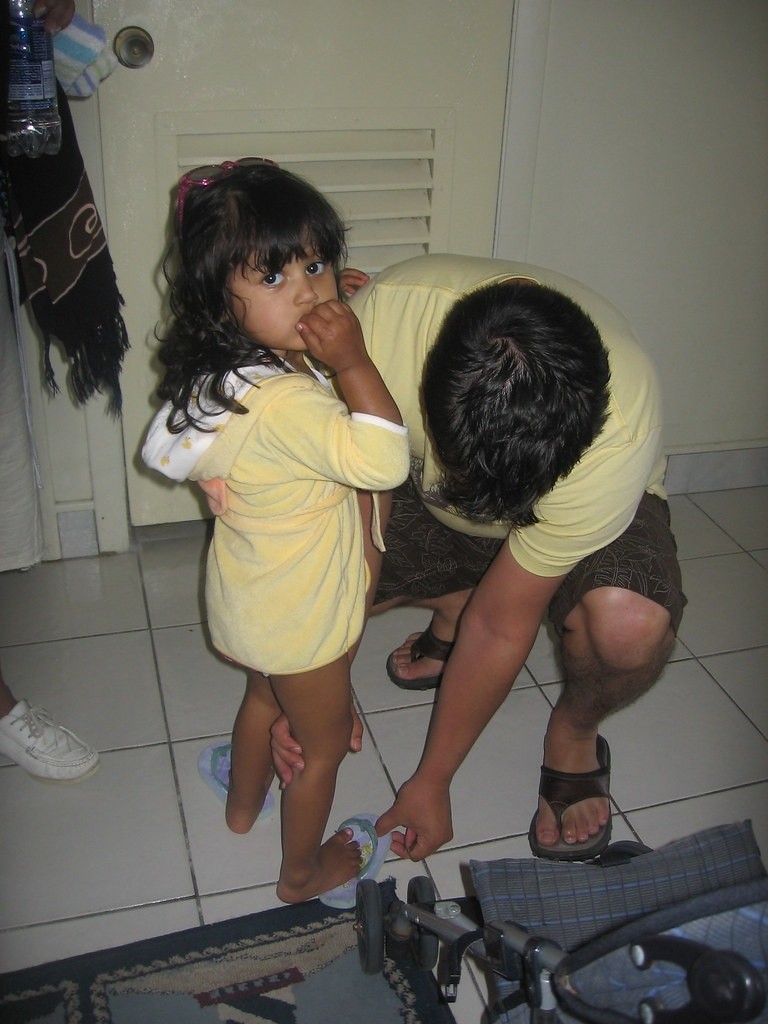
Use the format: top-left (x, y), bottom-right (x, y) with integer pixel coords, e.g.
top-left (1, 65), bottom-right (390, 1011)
top-left (350, 820), bottom-right (768, 1024)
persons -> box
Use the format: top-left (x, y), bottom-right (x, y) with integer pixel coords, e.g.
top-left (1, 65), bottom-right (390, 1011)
top-left (0, 671), bottom-right (102, 785)
top-left (270, 253), bottom-right (689, 862)
top-left (138, 158), bottom-right (411, 905)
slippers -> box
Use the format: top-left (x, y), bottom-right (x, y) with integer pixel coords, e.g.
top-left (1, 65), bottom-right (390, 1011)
top-left (319, 813), bottom-right (392, 908)
top-left (529, 731), bottom-right (611, 862)
top-left (387, 621), bottom-right (454, 690)
top-left (200, 742), bottom-right (273, 815)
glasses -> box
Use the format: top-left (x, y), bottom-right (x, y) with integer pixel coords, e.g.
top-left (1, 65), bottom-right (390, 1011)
top-left (178, 156), bottom-right (279, 242)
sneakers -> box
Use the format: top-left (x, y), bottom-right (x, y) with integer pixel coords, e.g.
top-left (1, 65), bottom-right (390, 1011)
top-left (0, 700), bottom-right (99, 785)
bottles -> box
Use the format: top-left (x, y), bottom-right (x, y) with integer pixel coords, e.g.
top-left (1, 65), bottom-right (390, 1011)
top-left (4, 0), bottom-right (62, 160)
